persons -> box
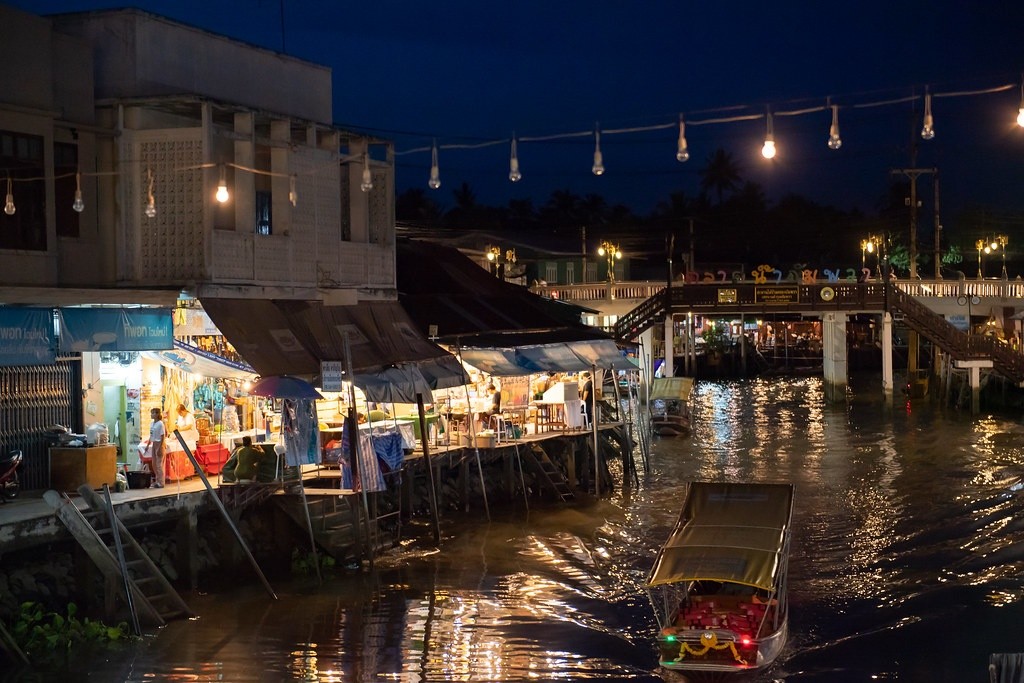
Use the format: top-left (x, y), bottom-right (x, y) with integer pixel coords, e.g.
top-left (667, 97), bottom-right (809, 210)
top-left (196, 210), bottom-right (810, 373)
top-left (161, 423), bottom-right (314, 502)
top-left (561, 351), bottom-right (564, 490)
top-left (145, 408), bottom-right (167, 488)
top-left (233, 436), bottom-right (265, 485)
top-left (175, 404), bottom-right (196, 440)
top-left (482, 384), bottom-right (503, 430)
top-left (582, 372), bottom-right (593, 428)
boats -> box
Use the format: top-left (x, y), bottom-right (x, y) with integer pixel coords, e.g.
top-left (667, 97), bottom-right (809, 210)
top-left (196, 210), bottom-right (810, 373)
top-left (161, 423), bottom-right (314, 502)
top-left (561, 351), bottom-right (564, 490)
top-left (618, 357), bottom-right (679, 395)
top-left (649, 376), bottom-right (694, 436)
top-left (643, 479), bottom-right (799, 672)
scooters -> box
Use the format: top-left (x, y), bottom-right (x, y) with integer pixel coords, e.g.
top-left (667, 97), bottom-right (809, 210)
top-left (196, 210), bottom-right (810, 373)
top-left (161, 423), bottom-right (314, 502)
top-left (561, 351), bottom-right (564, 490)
top-left (0, 448), bottom-right (23, 499)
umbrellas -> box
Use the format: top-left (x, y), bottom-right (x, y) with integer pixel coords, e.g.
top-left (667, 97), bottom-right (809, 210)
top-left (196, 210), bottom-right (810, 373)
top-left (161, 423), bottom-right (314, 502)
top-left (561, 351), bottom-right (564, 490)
top-left (246, 375), bottom-right (326, 435)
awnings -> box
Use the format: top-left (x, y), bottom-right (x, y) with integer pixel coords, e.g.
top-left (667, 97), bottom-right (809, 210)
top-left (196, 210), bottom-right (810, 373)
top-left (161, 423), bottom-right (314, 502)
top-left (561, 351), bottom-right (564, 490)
top-left (195, 292), bottom-right (472, 402)
top-left (456, 338), bottom-right (641, 376)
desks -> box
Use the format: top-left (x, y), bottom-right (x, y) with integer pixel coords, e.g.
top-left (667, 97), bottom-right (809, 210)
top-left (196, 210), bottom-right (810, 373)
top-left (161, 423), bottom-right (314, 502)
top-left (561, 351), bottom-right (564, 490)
top-left (392, 415), bottom-right (440, 446)
top-left (315, 420), bottom-right (415, 470)
top-left (139, 441), bottom-right (196, 484)
top-left (528, 400), bottom-right (581, 435)
top-left (214, 430), bottom-right (264, 456)
top-left (48, 444), bottom-right (117, 496)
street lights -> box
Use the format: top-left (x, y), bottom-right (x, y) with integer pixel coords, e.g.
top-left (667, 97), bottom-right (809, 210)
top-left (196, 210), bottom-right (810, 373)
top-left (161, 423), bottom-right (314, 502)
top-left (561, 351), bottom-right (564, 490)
top-left (598, 242), bottom-right (622, 284)
top-left (991, 233), bottom-right (1010, 281)
top-left (485, 243), bottom-right (501, 278)
top-left (977, 237), bottom-right (991, 281)
top-left (861, 235), bottom-right (883, 280)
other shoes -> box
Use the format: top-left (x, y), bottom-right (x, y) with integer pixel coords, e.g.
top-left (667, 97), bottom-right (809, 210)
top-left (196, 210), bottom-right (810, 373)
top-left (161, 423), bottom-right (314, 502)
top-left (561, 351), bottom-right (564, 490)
top-left (151, 483), bottom-right (164, 488)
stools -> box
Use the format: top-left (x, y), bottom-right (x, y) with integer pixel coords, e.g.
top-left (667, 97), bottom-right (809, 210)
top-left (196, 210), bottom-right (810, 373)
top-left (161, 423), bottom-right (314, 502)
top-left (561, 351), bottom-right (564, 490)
top-left (488, 414), bottom-right (508, 445)
